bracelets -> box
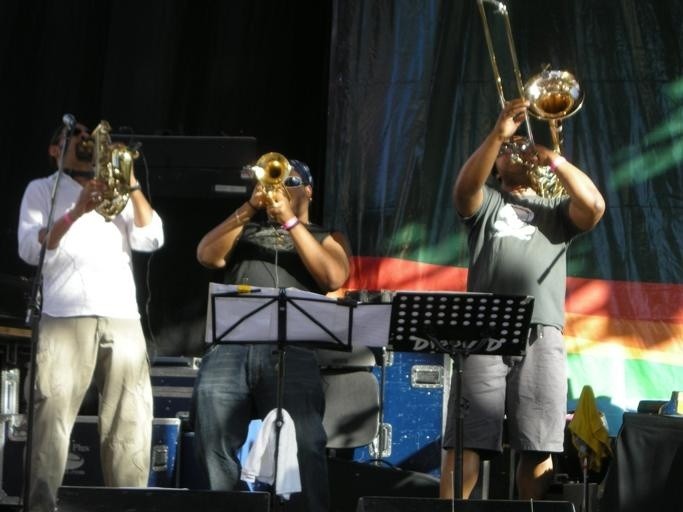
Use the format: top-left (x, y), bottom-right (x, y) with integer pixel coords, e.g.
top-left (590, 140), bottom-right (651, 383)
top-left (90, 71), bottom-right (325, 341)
top-left (549, 157), bottom-right (566, 172)
top-left (282, 216), bottom-right (298, 229)
top-left (63, 209), bottom-right (74, 225)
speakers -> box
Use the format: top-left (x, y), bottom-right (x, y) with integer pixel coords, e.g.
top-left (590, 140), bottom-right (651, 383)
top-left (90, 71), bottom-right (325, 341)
top-left (355, 496), bottom-right (575, 512)
top-left (54, 486), bottom-right (272, 512)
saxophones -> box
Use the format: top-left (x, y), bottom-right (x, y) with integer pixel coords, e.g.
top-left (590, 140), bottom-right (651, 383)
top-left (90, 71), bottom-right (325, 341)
top-left (80, 121), bottom-right (140, 220)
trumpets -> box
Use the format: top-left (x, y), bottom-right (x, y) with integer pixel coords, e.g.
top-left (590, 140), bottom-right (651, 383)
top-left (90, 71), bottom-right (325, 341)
top-left (254, 151), bottom-right (293, 222)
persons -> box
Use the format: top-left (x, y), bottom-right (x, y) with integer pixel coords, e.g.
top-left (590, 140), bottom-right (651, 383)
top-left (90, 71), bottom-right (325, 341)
top-left (15, 120), bottom-right (165, 512)
top-left (439, 96), bottom-right (609, 502)
top-left (193, 156), bottom-right (349, 512)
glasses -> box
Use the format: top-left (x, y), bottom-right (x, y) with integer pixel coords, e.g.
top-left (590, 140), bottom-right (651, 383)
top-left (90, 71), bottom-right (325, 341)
top-left (285, 176), bottom-right (303, 187)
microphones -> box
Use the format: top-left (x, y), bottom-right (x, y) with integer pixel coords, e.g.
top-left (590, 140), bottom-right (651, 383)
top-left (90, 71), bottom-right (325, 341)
top-left (63, 114), bottom-right (81, 136)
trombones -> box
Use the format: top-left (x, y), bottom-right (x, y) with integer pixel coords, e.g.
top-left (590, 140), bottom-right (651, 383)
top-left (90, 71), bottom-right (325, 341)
top-left (479, 0), bottom-right (585, 199)
